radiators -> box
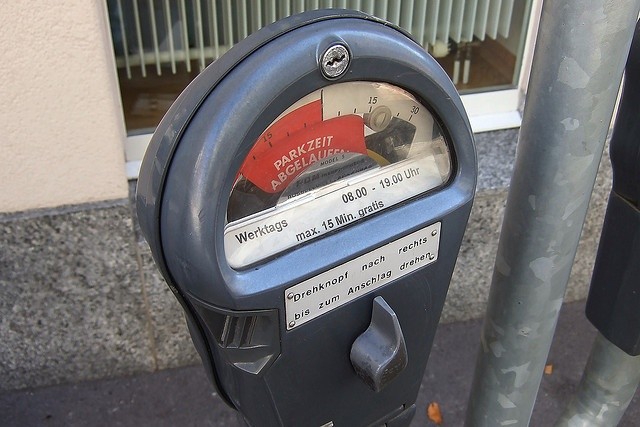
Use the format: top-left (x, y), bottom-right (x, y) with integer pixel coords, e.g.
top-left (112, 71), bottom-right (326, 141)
top-left (106, 0), bottom-right (516, 80)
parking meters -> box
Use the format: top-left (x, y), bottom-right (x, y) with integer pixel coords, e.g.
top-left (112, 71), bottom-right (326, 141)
top-left (136, 10), bottom-right (477, 426)
